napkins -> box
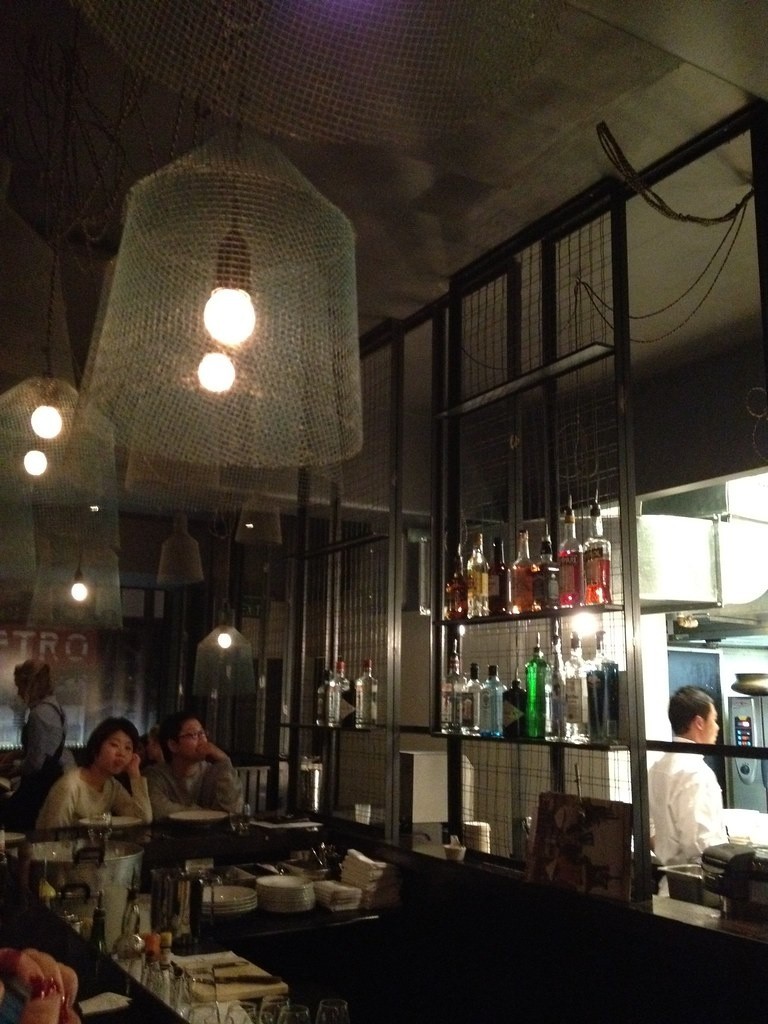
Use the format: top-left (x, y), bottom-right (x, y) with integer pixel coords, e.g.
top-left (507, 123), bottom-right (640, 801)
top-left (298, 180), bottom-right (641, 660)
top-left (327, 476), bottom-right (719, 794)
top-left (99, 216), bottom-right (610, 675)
top-left (314, 848), bottom-right (401, 911)
top-left (172, 950), bottom-right (288, 1002)
top-left (79, 992), bottom-right (132, 1014)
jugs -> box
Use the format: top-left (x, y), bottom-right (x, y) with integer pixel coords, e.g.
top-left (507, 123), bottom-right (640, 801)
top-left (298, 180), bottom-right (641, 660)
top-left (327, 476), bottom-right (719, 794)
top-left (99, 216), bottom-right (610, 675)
top-left (148, 868), bottom-right (214, 950)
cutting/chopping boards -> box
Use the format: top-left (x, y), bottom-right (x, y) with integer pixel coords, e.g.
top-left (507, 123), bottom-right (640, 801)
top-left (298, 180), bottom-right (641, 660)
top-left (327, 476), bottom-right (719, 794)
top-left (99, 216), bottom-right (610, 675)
top-left (174, 953), bottom-right (288, 1001)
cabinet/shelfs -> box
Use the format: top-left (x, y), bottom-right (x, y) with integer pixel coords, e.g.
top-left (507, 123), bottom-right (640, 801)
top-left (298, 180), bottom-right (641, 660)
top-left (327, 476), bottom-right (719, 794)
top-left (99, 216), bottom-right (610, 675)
top-left (436, 344), bottom-right (654, 899)
top-left (277, 534), bottom-right (389, 732)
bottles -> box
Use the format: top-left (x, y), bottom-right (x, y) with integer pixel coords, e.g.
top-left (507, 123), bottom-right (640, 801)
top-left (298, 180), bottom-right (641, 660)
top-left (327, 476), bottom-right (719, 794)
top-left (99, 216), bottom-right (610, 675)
top-left (127, 930), bottom-right (172, 1010)
top-left (441, 628), bottom-right (618, 744)
top-left (445, 501), bottom-right (611, 621)
top-left (89, 907), bottom-right (110, 954)
top-left (317, 657), bottom-right (379, 728)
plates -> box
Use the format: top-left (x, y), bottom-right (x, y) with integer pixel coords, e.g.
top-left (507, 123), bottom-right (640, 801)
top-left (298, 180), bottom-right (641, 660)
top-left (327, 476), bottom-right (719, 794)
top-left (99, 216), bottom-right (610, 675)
top-left (168, 811), bottom-right (227, 822)
top-left (202, 875), bottom-right (316, 919)
top-left (4, 831), bottom-right (28, 842)
top-left (79, 816), bottom-right (142, 826)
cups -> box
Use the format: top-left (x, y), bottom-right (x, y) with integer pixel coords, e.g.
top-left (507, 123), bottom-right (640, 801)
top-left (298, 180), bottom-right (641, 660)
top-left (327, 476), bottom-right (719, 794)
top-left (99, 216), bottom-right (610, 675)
top-left (444, 845), bottom-right (466, 862)
top-left (88, 810), bottom-right (114, 840)
top-left (180, 963), bottom-right (350, 1024)
top-left (230, 803), bottom-right (250, 831)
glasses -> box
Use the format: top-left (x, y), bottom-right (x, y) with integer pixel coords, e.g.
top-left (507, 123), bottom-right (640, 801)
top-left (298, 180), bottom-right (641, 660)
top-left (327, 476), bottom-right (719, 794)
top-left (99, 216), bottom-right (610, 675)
top-left (176, 729), bottom-right (209, 740)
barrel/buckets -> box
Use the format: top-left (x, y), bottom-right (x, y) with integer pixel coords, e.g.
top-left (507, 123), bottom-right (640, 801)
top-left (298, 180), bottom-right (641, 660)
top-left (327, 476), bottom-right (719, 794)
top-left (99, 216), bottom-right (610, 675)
top-left (29, 840), bottom-right (144, 951)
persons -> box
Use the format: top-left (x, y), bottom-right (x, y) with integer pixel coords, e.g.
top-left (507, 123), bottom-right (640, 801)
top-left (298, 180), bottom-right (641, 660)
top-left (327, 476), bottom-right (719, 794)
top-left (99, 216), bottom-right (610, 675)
top-left (147, 726), bottom-right (160, 761)
top-left (35, 717), bottom-right (153, 841)
top-left (142, 712), bottom-right (244, 821)
top-left (649, 687), bottom-right (729, 867)
top-left (0, 660), bottom-right (65, 832)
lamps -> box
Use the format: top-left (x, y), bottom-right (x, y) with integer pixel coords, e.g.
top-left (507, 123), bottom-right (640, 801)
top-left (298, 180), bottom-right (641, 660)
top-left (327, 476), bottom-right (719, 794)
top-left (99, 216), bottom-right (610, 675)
top-left (1, 0), bottom-right (364, 699)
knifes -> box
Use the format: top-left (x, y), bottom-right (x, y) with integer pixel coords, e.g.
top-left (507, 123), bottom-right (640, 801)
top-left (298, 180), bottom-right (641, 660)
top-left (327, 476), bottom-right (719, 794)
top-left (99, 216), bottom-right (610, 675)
top-left (197, 975), bottom-right (282, 985)
